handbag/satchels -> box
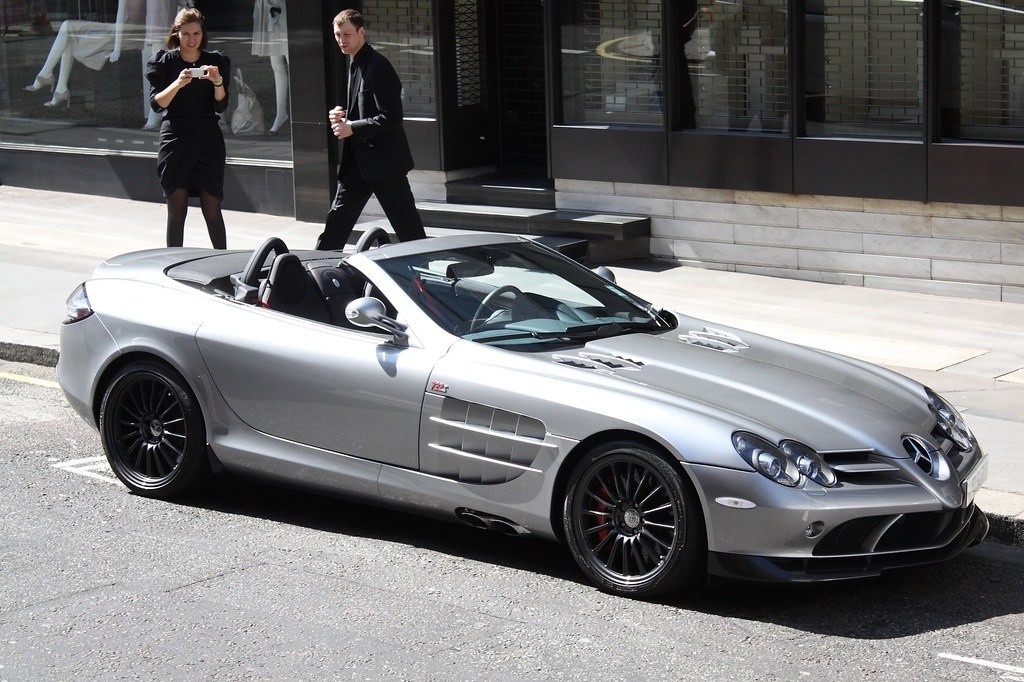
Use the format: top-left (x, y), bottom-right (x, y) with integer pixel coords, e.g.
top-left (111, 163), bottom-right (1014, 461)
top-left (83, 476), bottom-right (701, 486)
top-left (231, 69), bottom-right (264, 136)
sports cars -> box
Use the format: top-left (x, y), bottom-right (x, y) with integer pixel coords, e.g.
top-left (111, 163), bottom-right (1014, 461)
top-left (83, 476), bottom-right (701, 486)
top-left (55, 224), bottom-right (991, 599)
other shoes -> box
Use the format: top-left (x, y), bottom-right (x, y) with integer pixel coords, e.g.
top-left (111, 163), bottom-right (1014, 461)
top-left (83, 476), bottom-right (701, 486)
top-left (142, 122), bottom-right (161, 131)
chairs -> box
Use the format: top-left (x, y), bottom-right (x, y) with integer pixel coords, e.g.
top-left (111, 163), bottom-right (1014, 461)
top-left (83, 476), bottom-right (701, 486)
top-left (242, 236), bottom-right (333, 328)
top-left (340, 227), bottom-right (418, 316)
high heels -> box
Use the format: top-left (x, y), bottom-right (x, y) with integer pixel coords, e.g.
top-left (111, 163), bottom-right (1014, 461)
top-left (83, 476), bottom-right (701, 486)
top-left (43, 88), bottom-right (71, 109)
top-left (24, 74), bottom-right (55, 92)
top-left (266, 114), bottom-right (289, 137)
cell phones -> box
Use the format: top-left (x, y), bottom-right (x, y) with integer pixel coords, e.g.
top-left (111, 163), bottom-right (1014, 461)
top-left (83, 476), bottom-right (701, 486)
top-left (189, 68), bottom-right (204, 77)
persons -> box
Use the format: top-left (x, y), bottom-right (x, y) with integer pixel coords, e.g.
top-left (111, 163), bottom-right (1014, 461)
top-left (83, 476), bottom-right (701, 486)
top-left (314, 9), bottom-right (427, 253)
top-left (22, 0), bottom-right (146, 108)
top-left (714, 0), bottom-right (791, 134)
top-left (140, 0), bottom-right (195, 130)
top-left (251, 0), bottom-right (290, 137)
top-left (144, 6), bottom-right (230, 250)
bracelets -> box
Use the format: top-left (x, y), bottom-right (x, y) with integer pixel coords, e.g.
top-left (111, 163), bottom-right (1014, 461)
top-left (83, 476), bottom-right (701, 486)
top-left (212, 76), bottom-right (224, 87)
